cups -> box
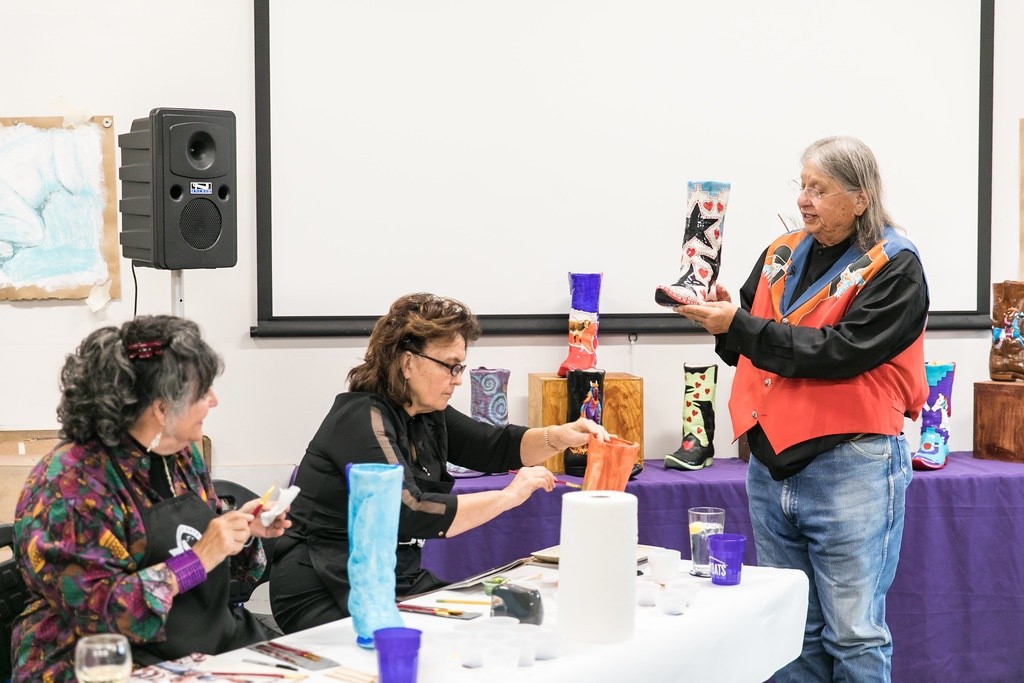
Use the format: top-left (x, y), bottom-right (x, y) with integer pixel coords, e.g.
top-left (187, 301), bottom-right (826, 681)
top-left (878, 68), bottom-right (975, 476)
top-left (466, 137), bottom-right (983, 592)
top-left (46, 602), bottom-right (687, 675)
top-left (373, 628), bottom-right (422, 682)
top-left (687, 506), bottom-right (726, 578)
top-left (74, 633), bottom-right (132, 683)
top-left (482, 575), bottom-right (512, 596)
top-left (707, 533), bottom-right (748, 585)
top-left (647, 549), bottom-right (681, 582)
top-left (451, 614), bottom-right (561, 682)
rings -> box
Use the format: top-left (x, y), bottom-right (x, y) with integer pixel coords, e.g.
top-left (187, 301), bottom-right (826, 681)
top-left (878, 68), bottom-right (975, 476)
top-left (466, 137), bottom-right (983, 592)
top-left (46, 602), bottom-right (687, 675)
top-left (693, 320), bottom-right (702, 327)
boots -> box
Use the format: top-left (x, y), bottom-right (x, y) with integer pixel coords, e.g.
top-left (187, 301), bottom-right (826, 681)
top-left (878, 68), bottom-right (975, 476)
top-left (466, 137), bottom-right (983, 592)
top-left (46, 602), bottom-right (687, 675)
top-left (654, 179), bottom-right (731, 328)
top-left (563, 370), bottom-right (645, 479)
top-left (555, 271), bottom-right (603, 377)
top-left (579, 432), bottom-right (639, 491)
top-left (664, 365), bottom-right (716, 471)
top-left (346, 462), bottom-right (405, 649)
top-left (989, 281), bottom-right (1024, 382)
top-left (910, 360), bottom-right (955, 470)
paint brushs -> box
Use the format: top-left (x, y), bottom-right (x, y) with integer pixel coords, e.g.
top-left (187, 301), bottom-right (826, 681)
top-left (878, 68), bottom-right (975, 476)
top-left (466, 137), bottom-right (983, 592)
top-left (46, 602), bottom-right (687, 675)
top-left (508, 470), bottom-right (581, 488)
top-left (211, 672), bottom-right (308, 683)
top-left (256, 643), bottom-right (321, 667)
top-left (398, 600), bottom-right (492, 616)
top-left (241, 659), bottom-right (298, 672)
top-left (248, 485), bottom-right (275, 526)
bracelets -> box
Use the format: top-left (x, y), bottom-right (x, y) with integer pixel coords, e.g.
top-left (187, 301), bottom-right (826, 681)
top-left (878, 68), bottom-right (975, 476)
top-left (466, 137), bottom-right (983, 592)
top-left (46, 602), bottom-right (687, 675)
top-left (545, 425), bottom-right (565, 453)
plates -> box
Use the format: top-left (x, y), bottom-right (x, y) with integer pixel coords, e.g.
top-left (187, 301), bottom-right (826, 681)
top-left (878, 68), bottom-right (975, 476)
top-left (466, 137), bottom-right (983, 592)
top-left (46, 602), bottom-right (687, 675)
top-left (530, 543), bottom-right (666, 565)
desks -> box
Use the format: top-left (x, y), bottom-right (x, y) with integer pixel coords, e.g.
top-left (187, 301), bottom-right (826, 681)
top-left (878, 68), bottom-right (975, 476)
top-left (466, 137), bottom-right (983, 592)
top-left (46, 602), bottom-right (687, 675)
top-left (65, 540), bottom-right (813, 683)
top-left (416, 445), bottom-right (1024, 682)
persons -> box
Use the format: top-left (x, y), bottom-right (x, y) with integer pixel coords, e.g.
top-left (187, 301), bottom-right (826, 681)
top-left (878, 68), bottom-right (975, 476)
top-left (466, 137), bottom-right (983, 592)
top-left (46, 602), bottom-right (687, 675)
top-left (14, 315), bottom-right (291, 683)
top-left (268, 293), bottom-right (619, 634)
top-left (677, 135), bottom-right (929, 683)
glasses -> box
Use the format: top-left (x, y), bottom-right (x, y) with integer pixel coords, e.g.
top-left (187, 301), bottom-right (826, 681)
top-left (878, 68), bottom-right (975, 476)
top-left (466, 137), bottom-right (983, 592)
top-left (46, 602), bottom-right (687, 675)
top-left (789, 175), bottom-right (862, 200)
top-left (411, 349), bottom-right (466, 377)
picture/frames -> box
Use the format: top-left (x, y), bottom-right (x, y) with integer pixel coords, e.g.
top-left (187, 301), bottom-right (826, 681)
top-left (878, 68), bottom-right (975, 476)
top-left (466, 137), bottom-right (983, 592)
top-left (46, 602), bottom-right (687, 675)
top-left (0, 112), bottom-right (120, 300)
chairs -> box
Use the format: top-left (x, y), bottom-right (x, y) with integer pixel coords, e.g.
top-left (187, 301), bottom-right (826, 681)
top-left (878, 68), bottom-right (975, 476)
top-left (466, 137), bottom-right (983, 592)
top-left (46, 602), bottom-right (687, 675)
top-left (0, 522), bottom-right (30, 683)
top-left (213, 480), bottom-right (284, 638)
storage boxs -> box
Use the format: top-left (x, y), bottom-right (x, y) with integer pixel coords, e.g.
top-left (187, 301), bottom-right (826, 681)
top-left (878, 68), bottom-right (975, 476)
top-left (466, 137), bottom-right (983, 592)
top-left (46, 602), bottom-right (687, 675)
top-left (528, 369), bottom-right (642, 479)
top-left (973, 380), bottom-right (1024, 462)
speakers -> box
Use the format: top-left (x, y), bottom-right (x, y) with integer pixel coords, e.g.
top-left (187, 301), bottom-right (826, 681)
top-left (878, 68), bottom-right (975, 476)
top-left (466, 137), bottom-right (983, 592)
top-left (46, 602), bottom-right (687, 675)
top-left (118, 107), bottom-right (238, 270)
